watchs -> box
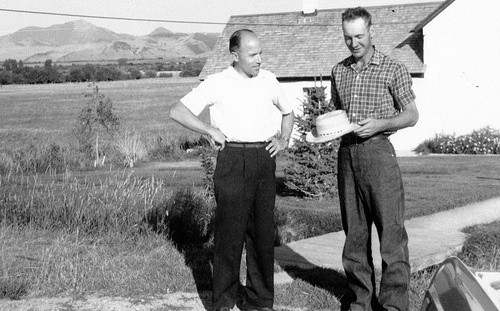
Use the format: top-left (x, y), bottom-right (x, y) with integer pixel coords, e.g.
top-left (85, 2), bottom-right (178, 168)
top-left (279, 135), bottom-right (289, 141)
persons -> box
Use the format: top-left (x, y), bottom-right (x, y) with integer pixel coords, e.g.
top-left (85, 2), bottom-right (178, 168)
top-left (170, 29), bottom-right (294, 311)
top-left (325, 7), bottom-right (419, 310)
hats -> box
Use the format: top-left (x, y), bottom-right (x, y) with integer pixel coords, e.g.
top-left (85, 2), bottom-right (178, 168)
top-left (306, 109), bottom-right (361, 142)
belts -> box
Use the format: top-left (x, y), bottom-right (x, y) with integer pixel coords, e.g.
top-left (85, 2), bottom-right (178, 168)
top-left (224, 142), bottom-right (270, 147)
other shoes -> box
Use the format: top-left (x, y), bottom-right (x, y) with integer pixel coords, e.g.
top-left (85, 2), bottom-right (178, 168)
top-left (215, 304), bottom-right (272, 310)
